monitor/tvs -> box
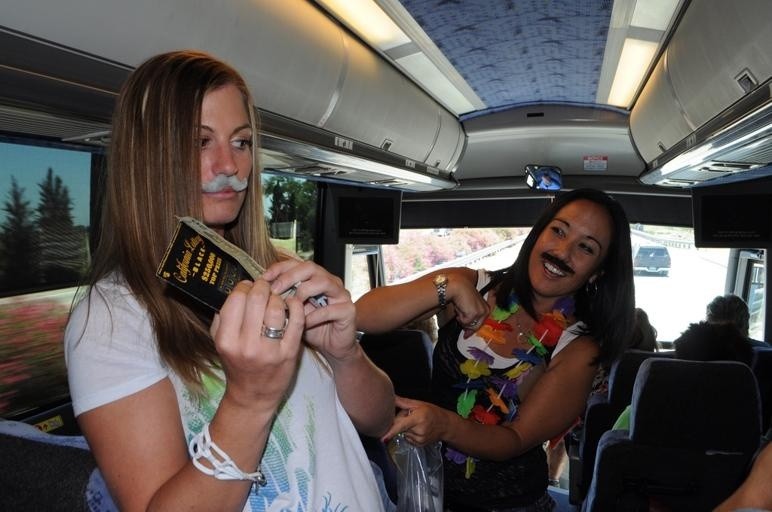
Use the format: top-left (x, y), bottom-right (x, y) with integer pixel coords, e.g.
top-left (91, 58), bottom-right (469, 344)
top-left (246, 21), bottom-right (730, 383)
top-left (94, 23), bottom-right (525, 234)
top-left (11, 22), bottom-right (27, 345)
top-left (691, 166), bottom-right (771, 249)
top-left (334, 189), bottom-right (402, 245)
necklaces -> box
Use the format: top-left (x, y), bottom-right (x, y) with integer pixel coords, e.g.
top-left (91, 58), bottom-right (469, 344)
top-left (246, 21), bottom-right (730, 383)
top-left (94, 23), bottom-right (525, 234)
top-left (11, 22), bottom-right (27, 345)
top-left (516, 306), bottom-right (534, 344)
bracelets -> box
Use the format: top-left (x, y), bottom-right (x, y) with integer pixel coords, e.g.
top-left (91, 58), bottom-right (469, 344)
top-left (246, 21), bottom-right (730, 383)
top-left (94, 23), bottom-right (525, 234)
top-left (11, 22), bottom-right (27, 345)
top-left (189, 423), bottom-right (266, 487)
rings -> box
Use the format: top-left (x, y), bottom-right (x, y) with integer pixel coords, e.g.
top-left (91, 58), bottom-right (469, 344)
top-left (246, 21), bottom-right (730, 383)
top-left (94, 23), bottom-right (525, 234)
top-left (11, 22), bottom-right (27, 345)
top-left (262, 325), bottom-right (284, 339)
top-left (470, 320), bottom-right (478, 326)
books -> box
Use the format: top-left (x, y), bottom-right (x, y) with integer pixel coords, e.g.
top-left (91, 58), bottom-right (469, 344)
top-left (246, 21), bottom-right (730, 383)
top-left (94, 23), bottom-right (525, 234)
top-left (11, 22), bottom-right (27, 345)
top-left (155, 216), bottom-right (296, 314)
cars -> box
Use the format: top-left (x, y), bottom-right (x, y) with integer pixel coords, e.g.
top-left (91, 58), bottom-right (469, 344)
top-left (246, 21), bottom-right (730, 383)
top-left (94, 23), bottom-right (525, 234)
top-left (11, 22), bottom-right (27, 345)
top-left (633, 244), bottom-right (671, 276)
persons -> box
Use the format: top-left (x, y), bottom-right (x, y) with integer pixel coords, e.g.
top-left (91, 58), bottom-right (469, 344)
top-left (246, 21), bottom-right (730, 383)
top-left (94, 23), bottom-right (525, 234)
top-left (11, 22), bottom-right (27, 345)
top-left (612, 321), bottom-right (755, 431)
top-left (545, 308), bottom-right (657, 489)
top-left (714, 428), bottom-right (772, 512)
top-left (353, 188), bottom-right (634, 512)
top-left (707, 296), bottom-right (772, 348)
top-left (64, 50), bottom-right (395, 512)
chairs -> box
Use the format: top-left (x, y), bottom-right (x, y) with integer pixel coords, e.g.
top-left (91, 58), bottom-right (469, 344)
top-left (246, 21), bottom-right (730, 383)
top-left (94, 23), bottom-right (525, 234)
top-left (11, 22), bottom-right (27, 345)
top-left (567, 344), bottom-right (772, 512)
top-left (355, 329), bottom-right (435, 507)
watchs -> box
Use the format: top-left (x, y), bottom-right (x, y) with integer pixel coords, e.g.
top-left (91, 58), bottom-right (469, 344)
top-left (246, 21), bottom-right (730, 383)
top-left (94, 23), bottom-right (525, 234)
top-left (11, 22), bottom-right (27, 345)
top-left (432, 274), bottom-right (447, 310)
